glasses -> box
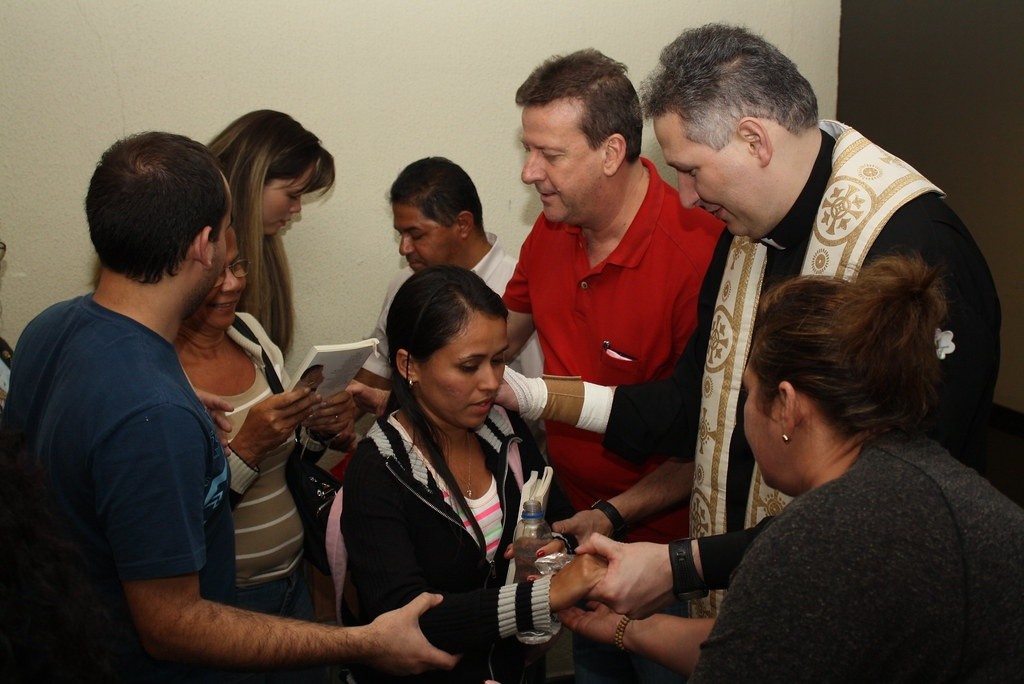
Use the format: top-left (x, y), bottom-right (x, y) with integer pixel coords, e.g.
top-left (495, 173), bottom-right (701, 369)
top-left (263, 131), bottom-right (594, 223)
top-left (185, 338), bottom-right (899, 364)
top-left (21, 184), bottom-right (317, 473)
top-left (213, 259), bottom-right (246, 287)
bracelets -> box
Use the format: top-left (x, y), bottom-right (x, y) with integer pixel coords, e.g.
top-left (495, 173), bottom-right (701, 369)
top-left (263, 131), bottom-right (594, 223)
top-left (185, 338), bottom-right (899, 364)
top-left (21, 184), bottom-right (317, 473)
top-left (668, 537), bottom-right (709, 603)
top-left (592, 500), bottom-right (630, 540)
top-left (614, 615), bottom-right (633, 650)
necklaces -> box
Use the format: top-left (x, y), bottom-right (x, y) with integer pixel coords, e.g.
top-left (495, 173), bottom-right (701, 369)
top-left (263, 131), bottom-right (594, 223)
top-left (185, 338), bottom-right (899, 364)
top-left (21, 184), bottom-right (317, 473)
top-left (456, 432), bottom-right (472, 498)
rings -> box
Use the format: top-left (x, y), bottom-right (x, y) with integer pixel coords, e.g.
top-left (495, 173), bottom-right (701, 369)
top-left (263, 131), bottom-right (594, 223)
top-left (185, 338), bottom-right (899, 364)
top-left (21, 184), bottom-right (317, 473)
top-left (335, 415), bottom-right (338, 422)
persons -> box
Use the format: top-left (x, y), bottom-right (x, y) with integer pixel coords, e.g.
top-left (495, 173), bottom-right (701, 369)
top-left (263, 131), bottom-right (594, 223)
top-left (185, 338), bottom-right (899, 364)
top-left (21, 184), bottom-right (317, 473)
top-left (293, 364), bottom-right (324, 392)
top-left (496, 21), bottom-right (1000, 616)
top-left (331, 158), bottom-right (546, 452)
top-left (503, 49), bottom-right (724, 551)
top-left (340, 264), bottom-right (611, 684)
top-left (555, 255), bottom-right (1024, 683)
top-left (0, 109), bottom-right (462, 684)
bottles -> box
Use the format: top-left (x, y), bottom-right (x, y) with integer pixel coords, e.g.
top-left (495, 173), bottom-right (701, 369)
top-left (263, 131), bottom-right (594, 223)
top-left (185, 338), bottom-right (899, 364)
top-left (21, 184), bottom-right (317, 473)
top-left (513, 500), bottom-right (553, 583)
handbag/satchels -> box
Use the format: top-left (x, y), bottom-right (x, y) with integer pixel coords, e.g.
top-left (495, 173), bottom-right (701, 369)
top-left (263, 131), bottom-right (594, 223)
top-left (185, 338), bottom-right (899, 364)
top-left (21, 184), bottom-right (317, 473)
top-left (285, 447), bottom-right (344, 576)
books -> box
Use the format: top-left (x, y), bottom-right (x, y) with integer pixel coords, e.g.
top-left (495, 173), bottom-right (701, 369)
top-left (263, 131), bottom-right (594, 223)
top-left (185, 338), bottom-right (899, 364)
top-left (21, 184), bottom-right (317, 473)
top-left (287, 338), bottom-right (379, 401)
